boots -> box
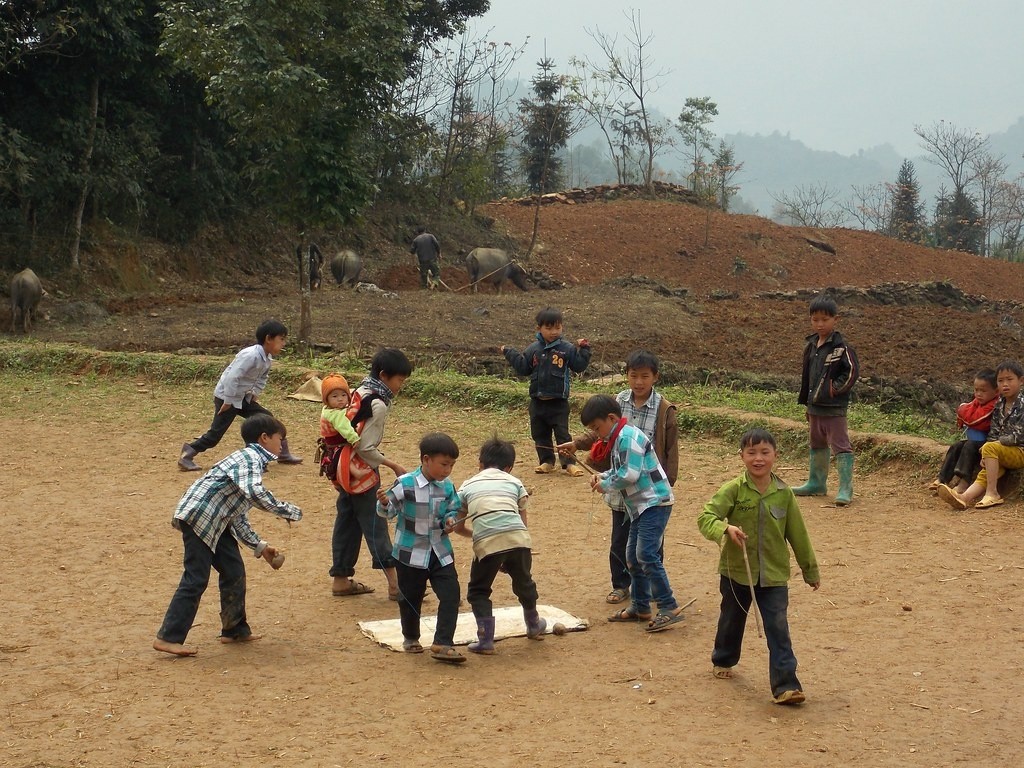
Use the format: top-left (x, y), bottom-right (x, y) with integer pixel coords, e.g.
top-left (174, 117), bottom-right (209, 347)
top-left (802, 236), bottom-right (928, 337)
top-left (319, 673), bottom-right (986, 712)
top-left (835, 453), bottom-right (854, 505)
top-left (468, 617), bottom-right (495, 654)
top-left (791, 448), bottom-right (831, 496)
top-left (523, 606), bottom-right (547, 639)
top-left (275, 439), bottom-right (304, 464)
top-left (178, 442), bottom-right (203, 470)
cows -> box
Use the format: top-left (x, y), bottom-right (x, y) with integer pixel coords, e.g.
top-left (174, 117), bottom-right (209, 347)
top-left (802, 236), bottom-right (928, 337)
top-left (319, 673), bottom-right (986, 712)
top-left (330, 250), bottom-right (369, 289)
top-left (465, 248), bottom-right (547, 291)
top-left (8, 266), bottom-right (45, 333)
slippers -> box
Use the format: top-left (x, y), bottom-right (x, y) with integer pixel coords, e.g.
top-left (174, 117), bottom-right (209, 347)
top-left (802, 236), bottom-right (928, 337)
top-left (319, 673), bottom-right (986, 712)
top-left (534, 463), bottom-right (556, 473)
top-left (645, 610), bottom-right (685, 631)
top-left (937, 484), bottom-right (966, 510)
top-left (388, 591), bottom-right (429, 601)
top-left (403, 639), bottom-right (424, 653)
top-left (332, 580), bottom-right (376, 596)
top-left (430, 644), bottom-right (467, 662)
top-left (975, 495), bottom-right (1004, 507)
top-left (607, 608), bottom-right (653, 622)
top-left (566, 465), bottom-right (584, 477)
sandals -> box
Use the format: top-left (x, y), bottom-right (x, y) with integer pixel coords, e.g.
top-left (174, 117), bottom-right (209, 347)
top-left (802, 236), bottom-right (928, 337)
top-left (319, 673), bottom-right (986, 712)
top-left (928, 481), bottom-right (946, 489)
top-left (953, 486), bottom-right (966, 493)
top-left (606, 587), bottom-right (629, 603)
top-left (774, 691), bottom-right (805, 704)
top-left (713, 665), bottom-right (732, 678)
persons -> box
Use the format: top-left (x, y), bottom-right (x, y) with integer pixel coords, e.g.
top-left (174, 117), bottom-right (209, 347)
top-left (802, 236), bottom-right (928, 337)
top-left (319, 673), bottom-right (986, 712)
top-left (297, 232), bottom-right (323, 290)
top-left (329, 349), bottom-right (429, 601)
top-left (790, 297), bottom-right (859, 504)
top-left (178, 319), bottom-right (303, 470)
top-left (556, 351), bottom-right (677, 603)
top-left (152, 414), bottom-right (302, 655)
top-left (501, 310), bottom-right (591, 476)
top-left (320, 374), bottom-right (370, 478)
top-left (581, 395), bottom-right (686, 632)
top-left (697, 429), bottom-right (820, 705)
top-left (375, 434), bottom-right (468, 662)
top-left (411, 226), bottom-right (442, 291)
top-left (456, 439), bottom-right (546, 650)
top-left (929, 368), bottom-right (1001, 495)
top-left (936, 361), bottom-right (1024, 510)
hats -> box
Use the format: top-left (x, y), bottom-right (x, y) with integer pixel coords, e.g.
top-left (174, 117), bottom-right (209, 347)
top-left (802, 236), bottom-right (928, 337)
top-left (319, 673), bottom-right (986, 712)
top-left (321, 373), bottom-right (352, 409)
